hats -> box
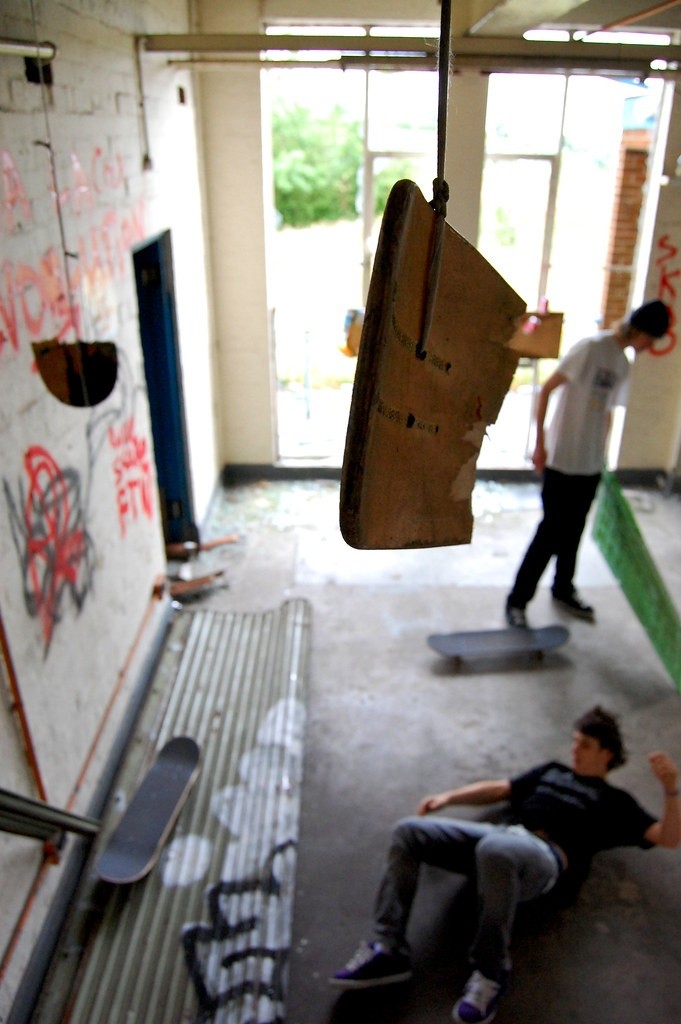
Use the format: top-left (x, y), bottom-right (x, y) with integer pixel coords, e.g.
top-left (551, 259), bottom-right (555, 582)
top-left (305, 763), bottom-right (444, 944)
top-left (630, 301), bottom-right (670, 338)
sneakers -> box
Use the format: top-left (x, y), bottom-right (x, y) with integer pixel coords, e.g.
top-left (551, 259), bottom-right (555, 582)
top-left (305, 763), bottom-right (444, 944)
top-left (328, 938), bottom-right (412, 989)
top-left (452, 958), bottom-right (514, 1024)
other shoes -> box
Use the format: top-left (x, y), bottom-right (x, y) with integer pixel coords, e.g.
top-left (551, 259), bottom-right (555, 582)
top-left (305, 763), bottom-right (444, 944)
top-left (553, 592), bottom-right (593, 618)
top-left (506, 605), bottom-right (526, 629)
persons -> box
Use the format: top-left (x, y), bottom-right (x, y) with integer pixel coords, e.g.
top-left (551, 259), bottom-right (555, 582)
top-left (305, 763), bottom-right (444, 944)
top-left (505, 298), bottom-right (670, 628)
top-left (327, 706), bottom-right (681, 1024)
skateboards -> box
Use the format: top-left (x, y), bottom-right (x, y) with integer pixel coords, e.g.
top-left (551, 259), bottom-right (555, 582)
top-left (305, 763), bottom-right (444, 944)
top-left (94, 733), bottom-right (204, 884)
top-left (426, 624), bottom-right (571, 671)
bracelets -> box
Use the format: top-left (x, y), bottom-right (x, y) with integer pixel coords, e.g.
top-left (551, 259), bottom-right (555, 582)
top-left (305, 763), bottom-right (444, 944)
top-left (665, 787), bottom-right (681, 797)
